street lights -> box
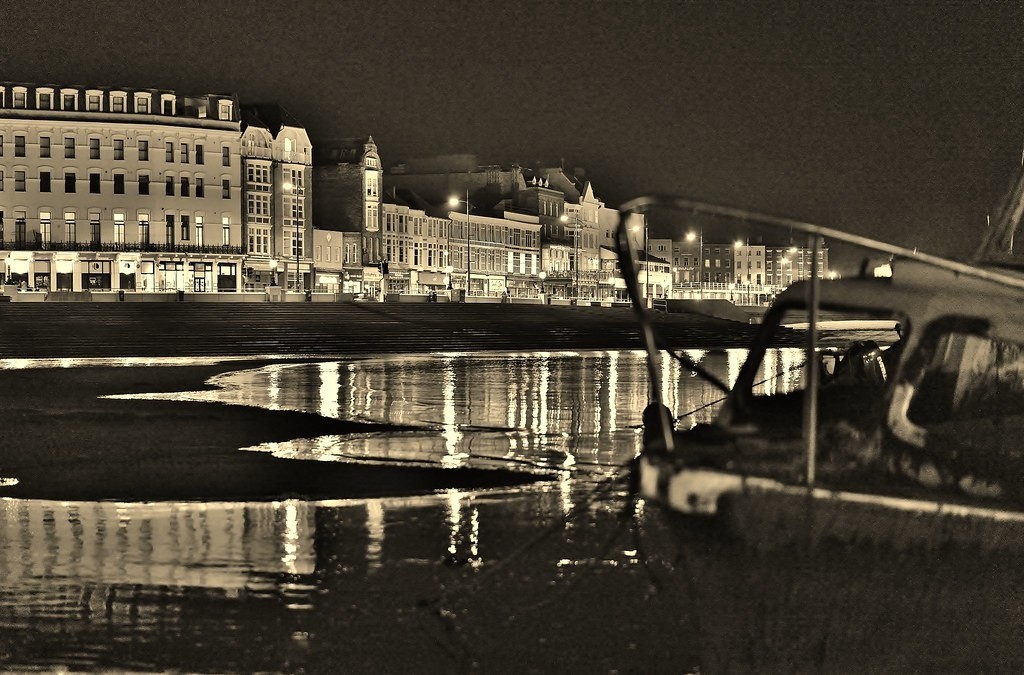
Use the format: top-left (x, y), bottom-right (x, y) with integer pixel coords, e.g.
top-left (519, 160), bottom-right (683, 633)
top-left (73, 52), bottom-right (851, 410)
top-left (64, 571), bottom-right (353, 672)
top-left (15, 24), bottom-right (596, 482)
top-left (631, 218), bottom-right (650, 296)
top-left (734, 232), bottom-right (752, 304)
top-left (779, 256), bottom-right (788, 288)
top-left (790, 242), bottom-right (805, 281)
top-left (283, 172), bottom-right (307, 293)
top-left (560, 212), bottom-right (586, 296)
top-left (448, 189), bottom-right (475, 297)
top-left (686, 226), bottom-right (703, 299)
top-left (268, 260), bottom-right (279, 287)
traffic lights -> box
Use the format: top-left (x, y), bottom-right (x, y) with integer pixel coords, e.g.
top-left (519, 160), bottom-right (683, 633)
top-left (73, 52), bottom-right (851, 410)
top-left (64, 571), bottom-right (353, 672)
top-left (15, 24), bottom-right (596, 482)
top-left (377, 262), bottom-right (382, 274)
top-left (382, 262), bottom-right (389, 275)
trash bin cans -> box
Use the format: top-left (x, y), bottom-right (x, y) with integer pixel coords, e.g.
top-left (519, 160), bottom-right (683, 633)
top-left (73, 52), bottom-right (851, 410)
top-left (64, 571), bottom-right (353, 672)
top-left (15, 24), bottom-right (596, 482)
top-left (304, 290), bottom-right (312, 301)
top-left (116, 290), bottom-right (124, 301)
top-left (547, 297), bottom-right (551, 304)
top-left (176, 290), bottom-right (185, 301)
top-left (501, 295), bottom-right (507, 303)
top-left (334, 293), bottom-right (353, 302)
top-left (386, 293), bottom-right (400, 302)
